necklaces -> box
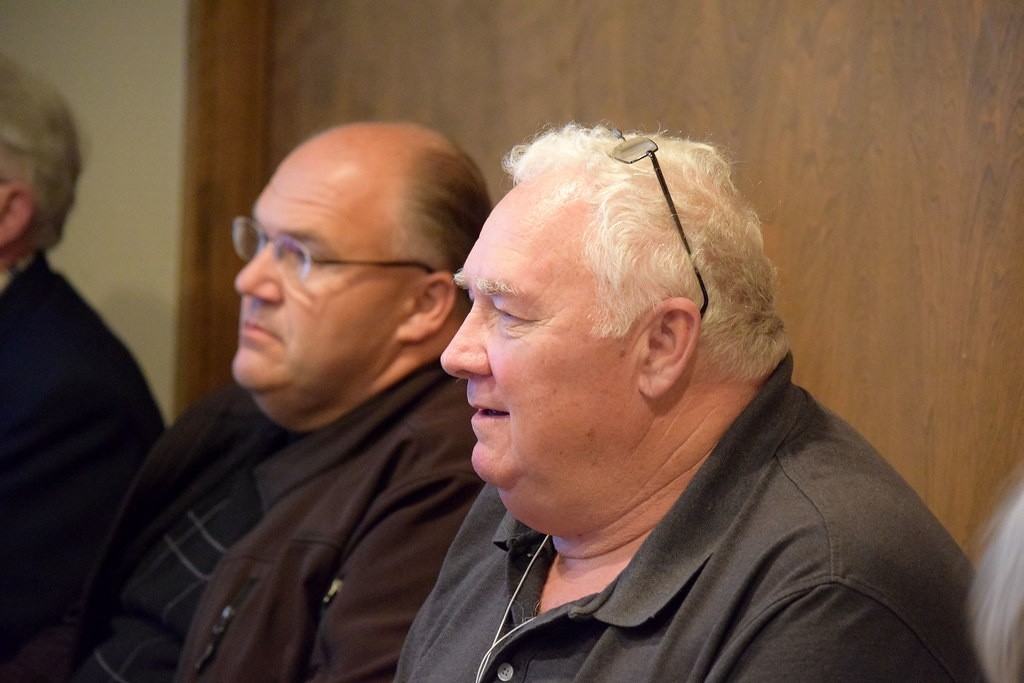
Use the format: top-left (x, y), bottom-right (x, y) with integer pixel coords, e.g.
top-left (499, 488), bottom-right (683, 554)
top-left (475, 534), bottom-right (549, 683)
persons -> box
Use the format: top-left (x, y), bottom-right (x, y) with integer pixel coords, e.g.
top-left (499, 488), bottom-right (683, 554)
top-left (70, 121), bottom-right (495, 683)
top-left (0, 55), bottom-right (165, 683)
top-left (391, 124), bottom-right (989, 683)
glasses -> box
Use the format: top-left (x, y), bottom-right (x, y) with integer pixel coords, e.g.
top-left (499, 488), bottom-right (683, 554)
top-left (608, 126), bottom-right (709, 320)
top-left (231, 215), bottom-right (434, 282)
